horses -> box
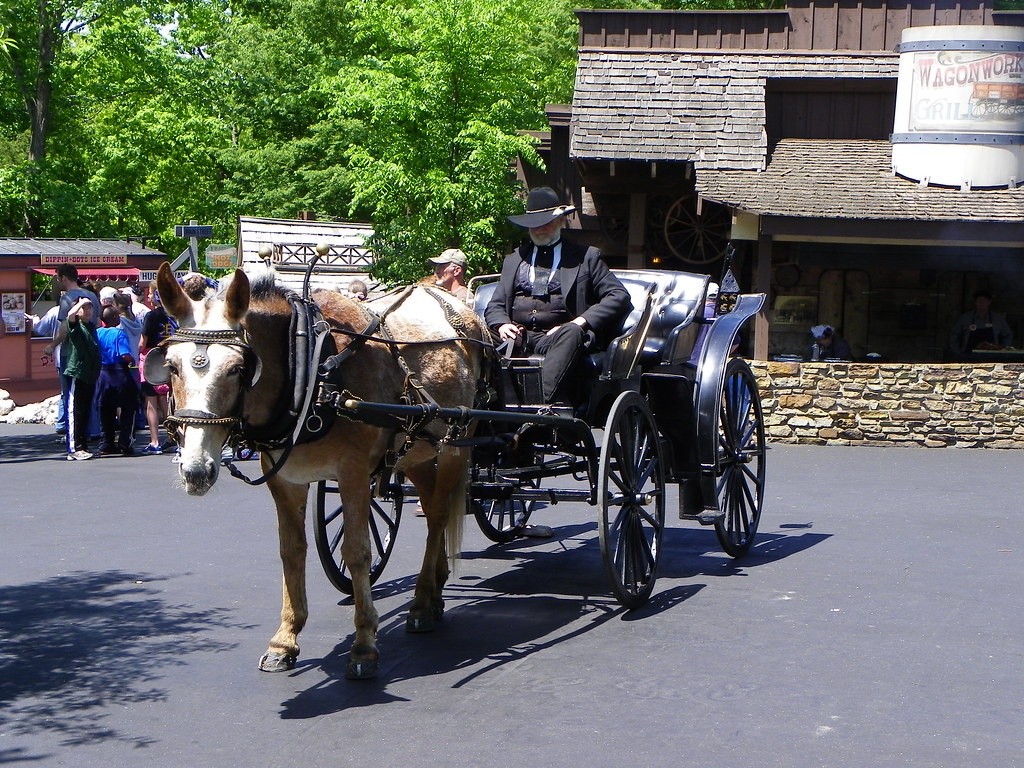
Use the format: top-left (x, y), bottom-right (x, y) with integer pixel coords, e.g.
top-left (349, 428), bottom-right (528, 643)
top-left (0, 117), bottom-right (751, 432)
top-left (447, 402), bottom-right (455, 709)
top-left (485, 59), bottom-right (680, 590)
top-left (153, 258), bottom-right (497, 682)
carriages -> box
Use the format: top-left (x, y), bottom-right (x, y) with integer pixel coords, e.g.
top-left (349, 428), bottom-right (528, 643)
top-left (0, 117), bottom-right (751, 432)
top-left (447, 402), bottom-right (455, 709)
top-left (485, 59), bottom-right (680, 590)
top-left (142, 241), bottom-right (769, 680)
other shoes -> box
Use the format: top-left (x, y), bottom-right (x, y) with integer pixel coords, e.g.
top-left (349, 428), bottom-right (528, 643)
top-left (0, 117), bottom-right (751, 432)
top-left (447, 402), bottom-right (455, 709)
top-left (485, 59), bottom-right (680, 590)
top-left (414, 507), bottom-right (426, 517)
top-left (66, 450), bottom-right (93, 461)
top-left (51, 435), bottom-right (67, 444)
top-left (221, 443), bottom-right (234, 460)
top-left (140, 443), bottom-right (163, 454)
top-left (234, 447), bottom-right (259, 460)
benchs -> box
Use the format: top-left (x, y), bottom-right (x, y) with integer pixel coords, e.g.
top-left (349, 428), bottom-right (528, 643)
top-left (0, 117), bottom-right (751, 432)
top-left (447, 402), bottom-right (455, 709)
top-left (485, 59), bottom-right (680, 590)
top-left (466, 269), bottom-right (711, 421)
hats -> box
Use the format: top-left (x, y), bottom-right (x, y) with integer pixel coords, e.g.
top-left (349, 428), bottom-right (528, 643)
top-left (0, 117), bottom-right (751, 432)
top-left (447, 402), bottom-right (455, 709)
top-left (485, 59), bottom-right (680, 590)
top-left (428, 248), bottom-right (468, 269)
top-left (507, 187), bottom-right (581, 228)
top-left (706, 282), bottom-right (719, 299)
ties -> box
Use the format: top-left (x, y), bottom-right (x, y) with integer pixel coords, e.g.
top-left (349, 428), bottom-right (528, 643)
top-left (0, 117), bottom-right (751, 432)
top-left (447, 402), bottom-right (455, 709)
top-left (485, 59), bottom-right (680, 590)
top-left (531, 238), bottom-right (561, 296)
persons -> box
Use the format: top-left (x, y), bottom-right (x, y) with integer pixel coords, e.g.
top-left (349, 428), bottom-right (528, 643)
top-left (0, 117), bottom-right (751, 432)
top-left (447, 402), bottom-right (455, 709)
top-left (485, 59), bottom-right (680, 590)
top-left (22, 262), bottom-right (263, 461)
top-left (434, 248), bottom-right (476, 314)
top-left (483, 186), bottom-right (632, 404)
top-left (810, 324), bottom-right (855, 361)
top-left (947, 289), bottom-right (1014, 362)
top-left (346, 279), bottom-right (371, 301)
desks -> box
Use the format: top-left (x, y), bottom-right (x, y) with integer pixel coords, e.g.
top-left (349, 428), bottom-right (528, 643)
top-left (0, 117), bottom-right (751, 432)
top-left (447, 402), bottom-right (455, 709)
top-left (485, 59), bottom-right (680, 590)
top-left (971, 349), bottom-right (1024, 363)
